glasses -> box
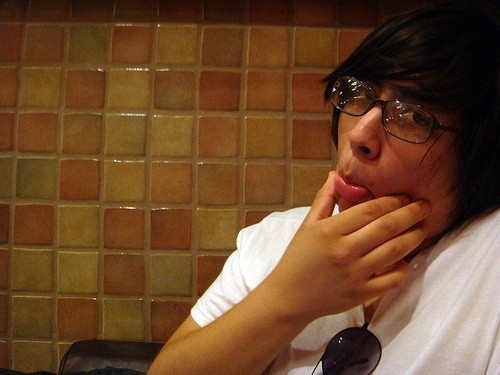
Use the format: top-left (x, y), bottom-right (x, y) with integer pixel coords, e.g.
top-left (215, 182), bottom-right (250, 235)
top-left (329, 72), bottom-right (460, 143)
top-left (309, 321), bottom-right (383, 375)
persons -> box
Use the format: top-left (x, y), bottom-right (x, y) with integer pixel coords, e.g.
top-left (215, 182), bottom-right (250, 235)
top-left (145, 0), bottom-right (500, 375)
